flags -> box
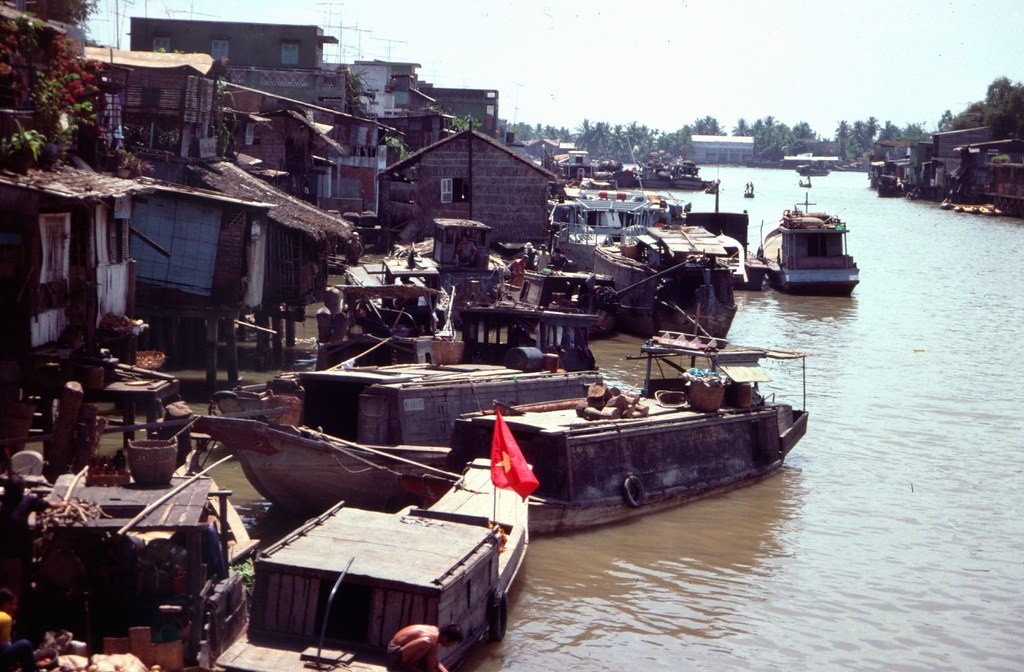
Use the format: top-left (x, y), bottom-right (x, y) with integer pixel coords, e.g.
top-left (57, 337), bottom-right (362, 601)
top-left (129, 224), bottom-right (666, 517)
top-left (490, 405), bottom-right (541, 503)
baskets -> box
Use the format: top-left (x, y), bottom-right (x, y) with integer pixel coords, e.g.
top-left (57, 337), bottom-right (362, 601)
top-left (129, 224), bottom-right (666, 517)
top-left (688, 380), bottom-right (725, 412)
top-left (136, 351), bottom-right (165, 369)
top-left (266, 395), bottom-right (302, 429)
top-left (433, 337), bottom-right (464, 366)
top-left (127, 435), bottom-right (178, 487)
top-left (85, 467), bottom-right (119, 487)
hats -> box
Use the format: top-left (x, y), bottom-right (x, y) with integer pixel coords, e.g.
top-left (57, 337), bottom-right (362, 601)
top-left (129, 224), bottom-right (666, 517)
top-left (539, 244), bottom-right (548, 250)
top-left (351, 232), bottom-right (359, 239)
top-left (524, 242), bottom-right (534, 248)
top-left (553, 248), bottom-right (560, 252)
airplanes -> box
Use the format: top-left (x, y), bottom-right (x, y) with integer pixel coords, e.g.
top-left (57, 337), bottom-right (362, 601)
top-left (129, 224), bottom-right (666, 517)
top-left (797, 165), bottom-right (830, 176)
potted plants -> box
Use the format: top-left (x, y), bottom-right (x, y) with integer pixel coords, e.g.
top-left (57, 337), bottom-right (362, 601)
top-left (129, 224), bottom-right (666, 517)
top-left (0, 128), bottom-right (48, 175)
top-left (117, 145), bottom-right (136, 179)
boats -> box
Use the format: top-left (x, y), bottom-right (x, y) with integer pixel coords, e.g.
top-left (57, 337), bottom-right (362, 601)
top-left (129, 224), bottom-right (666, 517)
top-left (799, 183), bottom-right (811, 187)
top-left (19, 447), bottom-right (247, 672)
top-left (614, 149), bottom-right (721, 193)
top-left (757, 192), bottom-right (860, 297)
top-left (213, 194), bottom-right (770, 447)
top-left (207, 459), bottom-right (533, 672)
top-left (744, 192), bottom-right (754, 198)
top-left (190, 305), bottom-right (810, 534)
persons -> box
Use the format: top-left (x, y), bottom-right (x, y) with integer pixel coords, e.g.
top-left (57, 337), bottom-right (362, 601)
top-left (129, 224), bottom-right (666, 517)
top-left (0, 586), bottom-right (36, 672)
top-left (507, 243), bottom-right (569, 281)
top-left (0, 485), bottom-right (68, 586)
top-left (453, 233), bottom-right (478, 269)
top-left (386, 623), bottom-right (465, 672)
top-left (577, 287), bottom-right (587, 310)
top-left (53, 325), bottom-right (104, 389)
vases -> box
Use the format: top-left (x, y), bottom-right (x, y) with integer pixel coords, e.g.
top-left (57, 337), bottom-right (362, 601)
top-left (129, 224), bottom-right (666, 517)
top-left (42, 142), bottom-right (64, 162)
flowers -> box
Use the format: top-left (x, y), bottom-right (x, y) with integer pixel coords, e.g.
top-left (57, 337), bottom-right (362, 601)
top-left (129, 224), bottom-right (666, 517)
top-left (29, 30), bottom-right (110, 142)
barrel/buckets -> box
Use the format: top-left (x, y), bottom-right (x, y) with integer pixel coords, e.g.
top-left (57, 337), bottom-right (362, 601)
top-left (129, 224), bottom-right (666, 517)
top-left (504, 347), bottom-right (543, 373)
